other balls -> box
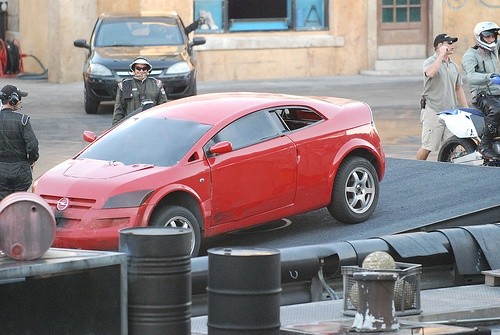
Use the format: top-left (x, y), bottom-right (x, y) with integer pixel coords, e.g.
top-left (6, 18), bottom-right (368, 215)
top-left (349, 251), bottom-right (415, 310)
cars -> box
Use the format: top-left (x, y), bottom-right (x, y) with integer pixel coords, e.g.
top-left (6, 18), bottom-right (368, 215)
top-left (26, 93), bottom-right (386, 257)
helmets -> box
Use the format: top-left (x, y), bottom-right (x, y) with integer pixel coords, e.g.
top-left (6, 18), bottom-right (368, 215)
top-left (473, 22), bottom-right (500, 51)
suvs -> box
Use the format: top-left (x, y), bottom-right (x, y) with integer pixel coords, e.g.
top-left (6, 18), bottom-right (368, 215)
top-left (74, 9), bottom-right (207, 115)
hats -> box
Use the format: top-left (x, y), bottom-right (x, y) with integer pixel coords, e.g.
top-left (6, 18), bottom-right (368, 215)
top-left (130, 56), bottom-right (152, 73)
top-left (0, 85), bottom-right (28, 100)
top-left (434, 34), bottom-right (458, 48)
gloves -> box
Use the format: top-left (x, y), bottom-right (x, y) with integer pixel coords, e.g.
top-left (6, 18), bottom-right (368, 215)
top-left (490, 74), bottom-right (500, 85)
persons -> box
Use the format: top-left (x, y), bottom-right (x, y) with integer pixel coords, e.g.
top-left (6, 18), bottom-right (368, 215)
top-left (461, 22), bottom-right (500, 159)
top-left (0, 85), bottom-right (39, 203)
top-left (416, 34), bottom-right (468, 163)
top-left (112, 56), bottom-right (167, 126)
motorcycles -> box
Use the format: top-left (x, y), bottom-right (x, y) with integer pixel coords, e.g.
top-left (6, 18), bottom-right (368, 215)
top-left (436, 77), bottom-right (500, 167)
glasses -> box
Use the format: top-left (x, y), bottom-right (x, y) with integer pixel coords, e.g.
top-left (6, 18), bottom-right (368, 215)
top-left (134, 66), bottom-right (147, 71)
top-left (442, 40), bottom-right (453, 45)
top-left (17, 95), bottom-right (21, 101)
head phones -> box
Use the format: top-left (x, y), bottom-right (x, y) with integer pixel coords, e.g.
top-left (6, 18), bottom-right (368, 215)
top-left (0, 92), bottom-right (19, 105)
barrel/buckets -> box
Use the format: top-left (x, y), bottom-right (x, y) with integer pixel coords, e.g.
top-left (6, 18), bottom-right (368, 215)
top-left (206, 246), bottom-right (282, 335)
top-left (117, 226), bottom-right (193, 335)
top-left (0, 191), bottom-right (57, 262)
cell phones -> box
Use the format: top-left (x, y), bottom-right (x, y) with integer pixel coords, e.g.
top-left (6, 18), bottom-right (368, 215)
top-left (421, 96), bottom-right (425, 107)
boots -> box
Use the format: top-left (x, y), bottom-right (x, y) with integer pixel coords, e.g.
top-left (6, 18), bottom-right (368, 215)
top-left (477, 135), bottom-right (500, 161)
